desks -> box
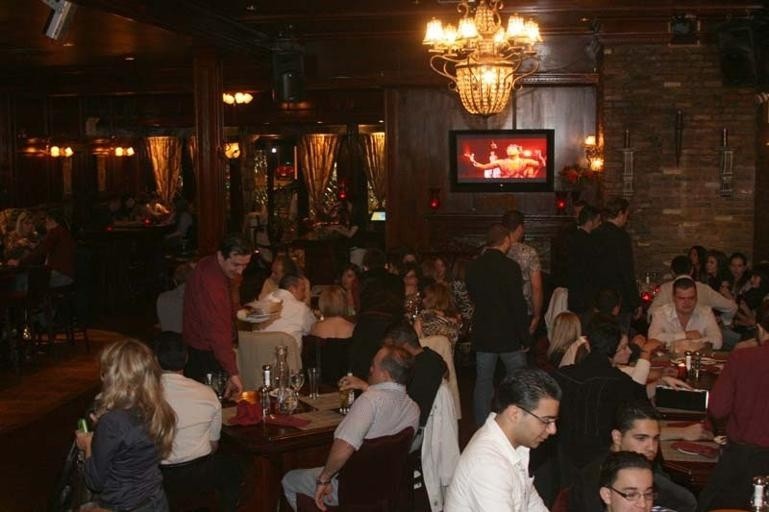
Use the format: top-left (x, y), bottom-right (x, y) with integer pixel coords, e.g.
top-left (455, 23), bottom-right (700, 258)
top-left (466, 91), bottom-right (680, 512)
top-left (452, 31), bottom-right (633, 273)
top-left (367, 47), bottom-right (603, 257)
top-left (104, 226), bottom-right (175, 318)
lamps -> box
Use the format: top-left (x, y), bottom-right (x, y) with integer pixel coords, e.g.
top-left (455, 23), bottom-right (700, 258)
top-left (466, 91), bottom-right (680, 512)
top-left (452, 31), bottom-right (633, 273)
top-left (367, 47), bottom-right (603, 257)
top-left (553, 190), bottom-right (569, 215)
top-left (421, 0), bottom-right (543, 118)
top-left (429, 187), bottom-right (440, 214)
top-left (583, 135), bottom-right (603, 178)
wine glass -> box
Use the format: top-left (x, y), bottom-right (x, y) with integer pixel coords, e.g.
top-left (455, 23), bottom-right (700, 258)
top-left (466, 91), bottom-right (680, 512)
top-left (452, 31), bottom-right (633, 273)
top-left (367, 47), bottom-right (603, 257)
top-left (272, 374), bottom-right (304, 417)
top-left (206, 371), bottom-right (228, 400)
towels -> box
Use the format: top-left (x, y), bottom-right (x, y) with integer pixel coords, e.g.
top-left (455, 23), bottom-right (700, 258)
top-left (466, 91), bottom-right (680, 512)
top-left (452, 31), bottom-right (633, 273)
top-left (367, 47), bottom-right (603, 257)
top-left (671, 439), bottom-right (717, 459)
top-left (226, 400), bottom-right (263, 425)
top-left (265, 412), bottom-right (311, 428)
top-left (660, 422), bottom-right (703, 441)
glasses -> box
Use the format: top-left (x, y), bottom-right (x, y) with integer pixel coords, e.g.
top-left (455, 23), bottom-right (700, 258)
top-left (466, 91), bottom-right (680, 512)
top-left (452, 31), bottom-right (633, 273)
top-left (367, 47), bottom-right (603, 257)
top-left (515, 405), bottom-right (560, 424)
top-left (605, 486), bottom-right (658, 501)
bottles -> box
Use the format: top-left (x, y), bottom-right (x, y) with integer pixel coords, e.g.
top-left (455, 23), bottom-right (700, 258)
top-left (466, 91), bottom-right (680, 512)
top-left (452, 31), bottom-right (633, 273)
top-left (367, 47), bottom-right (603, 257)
top-left (404, 291), bottom-right (423, 324)
top-left (258, 365), bottom-right (274, 417)
top-left (339, 378), bottom-right (354, 414)
top-left (671, 350), bottom-right (724, 378)
top-left (272, 344), bottom-right (290, 390)
top-left (640, 272), bottom-right (662, 315)
top-left (747, 477), bottom-right (769, 511)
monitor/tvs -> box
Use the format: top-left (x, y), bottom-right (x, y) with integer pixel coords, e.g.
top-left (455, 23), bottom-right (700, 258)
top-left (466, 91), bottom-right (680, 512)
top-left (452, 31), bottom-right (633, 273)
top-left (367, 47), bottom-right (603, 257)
top-left (449, 128), bottom-right (554, 193)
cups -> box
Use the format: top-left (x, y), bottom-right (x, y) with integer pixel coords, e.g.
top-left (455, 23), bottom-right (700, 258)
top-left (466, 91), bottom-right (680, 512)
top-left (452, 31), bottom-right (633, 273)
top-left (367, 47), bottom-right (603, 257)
top-left (78, 417), bottom-right (92, 432)
top-left (305, 365), bottom-right (321, 399)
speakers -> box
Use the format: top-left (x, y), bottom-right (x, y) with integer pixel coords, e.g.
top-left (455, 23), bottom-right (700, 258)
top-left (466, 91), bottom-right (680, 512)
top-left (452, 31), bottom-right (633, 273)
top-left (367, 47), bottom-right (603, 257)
top-left (718, 16), bottom-right (768, 87)
top-left (271, 51), bottom-right (305, 102)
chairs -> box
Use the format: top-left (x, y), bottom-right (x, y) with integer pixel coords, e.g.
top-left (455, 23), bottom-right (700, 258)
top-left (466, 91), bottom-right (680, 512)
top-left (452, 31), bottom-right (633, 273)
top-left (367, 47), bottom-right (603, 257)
top-left (295, 426), bottom-right (414, 511)
top-left (0, 264), bottom-right (92, 387)
top-left (551, 486), bottom-right (572, 511)
top-left (233, 330), bottom-right (303, 391)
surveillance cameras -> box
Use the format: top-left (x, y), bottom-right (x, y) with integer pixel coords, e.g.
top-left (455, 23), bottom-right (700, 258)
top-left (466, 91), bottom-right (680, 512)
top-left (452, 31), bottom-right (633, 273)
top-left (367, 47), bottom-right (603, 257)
top-left (41, 0), bottom-right (85, 44)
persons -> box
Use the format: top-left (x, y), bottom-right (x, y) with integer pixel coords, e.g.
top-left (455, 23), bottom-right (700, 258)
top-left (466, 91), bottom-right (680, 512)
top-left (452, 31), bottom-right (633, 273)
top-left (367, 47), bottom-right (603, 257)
top-left (1, 188), bottom-right (318, 511)
top-left (463, 144), bottom-right (547, 178)
top-left (280, 188), bottom-right (768, 511)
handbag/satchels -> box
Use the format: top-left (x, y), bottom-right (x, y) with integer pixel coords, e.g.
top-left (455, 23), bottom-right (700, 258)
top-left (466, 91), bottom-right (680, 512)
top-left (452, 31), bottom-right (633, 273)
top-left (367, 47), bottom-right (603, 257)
top-left (652, 384), bottom-right (709, 410)
top-left (48, 441), bottom-right (85, 512)
